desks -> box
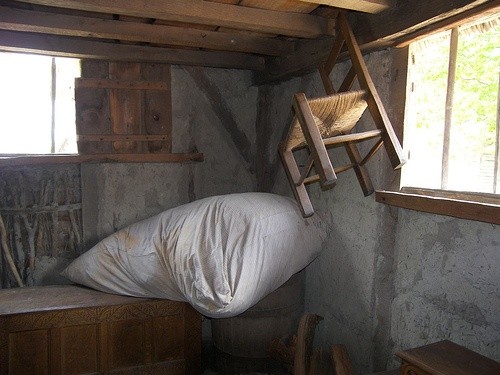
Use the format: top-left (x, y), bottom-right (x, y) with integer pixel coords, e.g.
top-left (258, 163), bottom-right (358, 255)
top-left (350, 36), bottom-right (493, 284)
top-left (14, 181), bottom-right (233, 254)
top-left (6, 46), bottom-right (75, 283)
top-left (0, 283), bottom-right (205, 375)
top-left (395, 339), bottom-right (500, 375)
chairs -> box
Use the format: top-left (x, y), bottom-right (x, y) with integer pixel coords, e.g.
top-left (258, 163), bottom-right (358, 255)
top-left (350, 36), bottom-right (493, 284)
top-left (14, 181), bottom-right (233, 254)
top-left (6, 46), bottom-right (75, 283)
top-left (278, 9), bottom-right (407, 218)
